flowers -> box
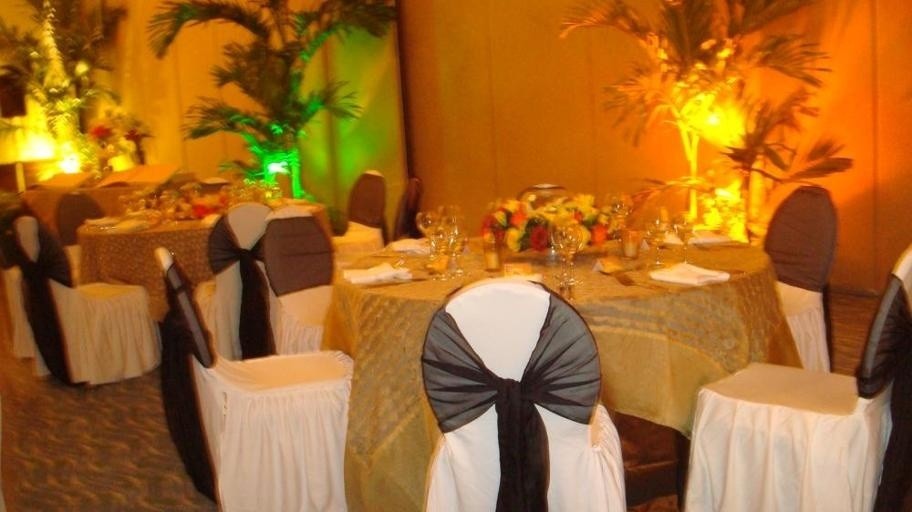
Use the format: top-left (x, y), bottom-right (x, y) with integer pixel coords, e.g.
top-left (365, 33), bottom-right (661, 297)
top-left (123, 116), bottom-right (153, 144)
top-left (84, 122), bottom-right (118, 168)
top-left (482, 192), bottom-right (614, 256)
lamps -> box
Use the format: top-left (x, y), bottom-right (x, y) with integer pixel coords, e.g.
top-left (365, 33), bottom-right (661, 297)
top-left (1, 115), bottom-right (55, 196)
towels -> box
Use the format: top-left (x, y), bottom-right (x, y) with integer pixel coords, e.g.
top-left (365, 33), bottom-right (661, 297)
top-left (646, 261), bottom-right (732, 286)
top-left (512, 273), bottom-right (544, 290)
top-left (389, 239), bottom-right (429, 253)
top-left (338, 262), bottom-right (412, 285)
top-left (83, 216), bottom-right (118, 227)
top-left (115, 218), bottom-right (149, 231)
top-left (693, 230), bottom-right (736, 247)
top-left (201, 213), bottom-right (221, 228)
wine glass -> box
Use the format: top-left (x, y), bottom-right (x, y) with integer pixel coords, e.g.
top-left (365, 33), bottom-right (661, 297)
top-left (117, 185), bottom-right (282, 222)
top-left (415, 192), bottom-right (693, 287)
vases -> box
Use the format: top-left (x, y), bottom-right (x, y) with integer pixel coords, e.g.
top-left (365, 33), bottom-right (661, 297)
top-left (97, 159), bottom-right (113, 178)
top-left (132, 142), bottom-right (144, 167)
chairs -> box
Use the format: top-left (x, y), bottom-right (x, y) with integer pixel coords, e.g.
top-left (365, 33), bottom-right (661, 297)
top-left (55, 192), bottom-right (103, 275)
top-left (682, 244), bottom-right (912, 512)
top-left (1, 209), bottom-right (81, 355)
top-left (393, 177), bottom-right (421, 247)
top-left (15, 216), bottom-right (161, 389)
top-left (263, 209), bottom-right (343, 353)
top-left (762, 186), bottom-right (837, 377)
top-left (202, 178), bottom-right (227, 195)
top-left (421, 280), bottom-right (626, 512)
top-left (329, 171), bottom-right (387, 259)
top-left (154, 246), bottom-right (356, 511)
top-left (196, 203), bottom-right (333, 358)
